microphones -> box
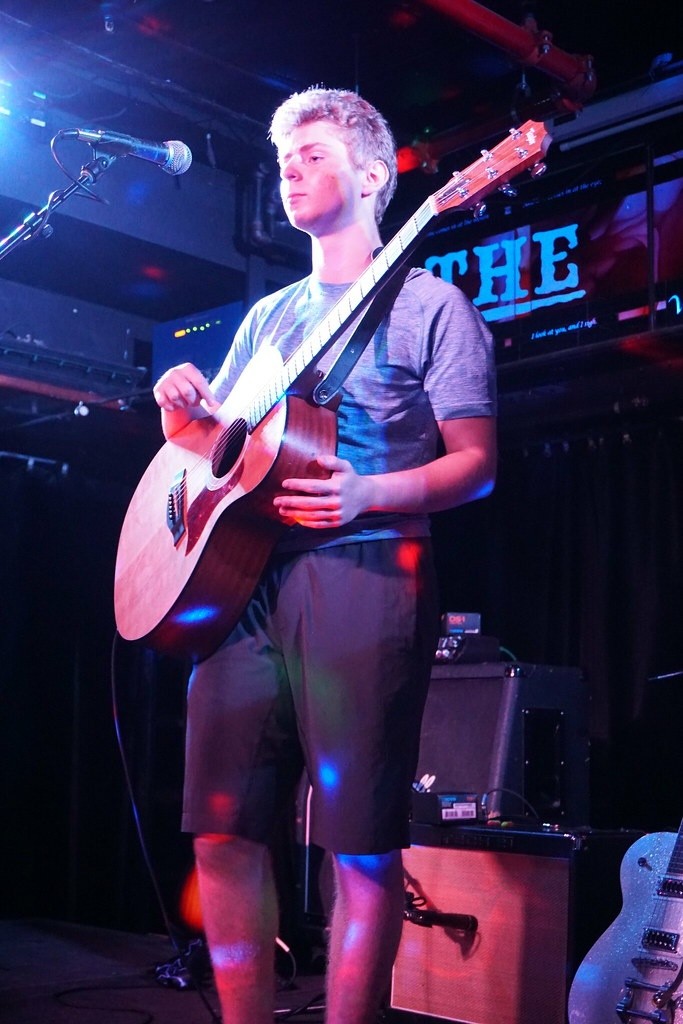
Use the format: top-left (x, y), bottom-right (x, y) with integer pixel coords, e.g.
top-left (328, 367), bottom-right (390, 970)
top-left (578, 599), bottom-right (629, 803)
top-left (404, 910), bottom-right (478, 933)
top-left (58, 127), bottom-right (192, 176)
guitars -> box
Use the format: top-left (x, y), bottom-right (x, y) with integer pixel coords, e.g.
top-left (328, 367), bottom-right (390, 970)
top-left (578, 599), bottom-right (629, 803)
top-left (108, 117), bottom-right (556, 671)
top-left (562, 817), bottom-right (682, 1023)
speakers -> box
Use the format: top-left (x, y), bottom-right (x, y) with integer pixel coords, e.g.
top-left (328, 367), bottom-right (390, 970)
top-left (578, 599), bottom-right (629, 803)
top-left (291, 662), bottom-right (650, 1024)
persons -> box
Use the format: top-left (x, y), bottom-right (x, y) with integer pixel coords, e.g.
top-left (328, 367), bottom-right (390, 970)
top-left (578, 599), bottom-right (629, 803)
top-left (153, 89), bottom-right (498, 1024)
